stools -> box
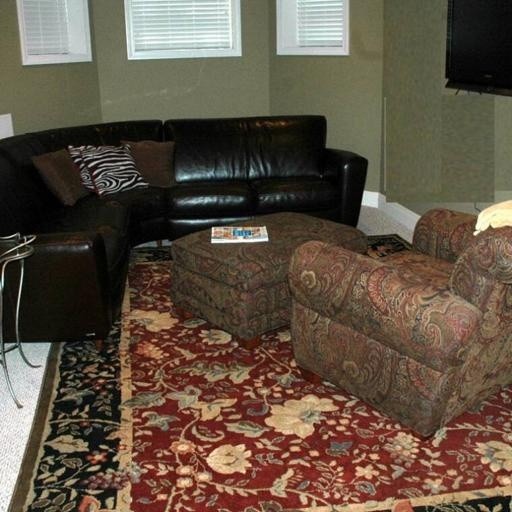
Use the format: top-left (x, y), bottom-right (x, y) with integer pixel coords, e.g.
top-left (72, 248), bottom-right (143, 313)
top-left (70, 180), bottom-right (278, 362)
top-left (172, 211), bottom-right (369, 340)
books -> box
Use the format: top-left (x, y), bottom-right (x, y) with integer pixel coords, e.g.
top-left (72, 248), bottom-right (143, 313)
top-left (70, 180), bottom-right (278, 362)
top-left (210, 226), bottom-right (269, 244)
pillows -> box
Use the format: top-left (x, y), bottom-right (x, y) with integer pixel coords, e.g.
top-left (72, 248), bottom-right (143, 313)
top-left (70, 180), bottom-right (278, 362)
top-left (80, 143), bottom-right (150, 199)
top-left (29, 148), bottom-right (90, 206)
top-left (68, 145), bottom-right (98, 193)
top-left (119, 140), bottom-right (175, 188)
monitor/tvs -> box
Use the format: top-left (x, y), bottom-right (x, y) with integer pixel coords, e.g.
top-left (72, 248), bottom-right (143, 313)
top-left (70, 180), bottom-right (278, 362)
top-left (444, 0), bottom-right (512, 98)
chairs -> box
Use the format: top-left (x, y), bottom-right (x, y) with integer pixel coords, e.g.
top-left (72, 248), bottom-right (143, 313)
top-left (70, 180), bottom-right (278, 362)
top-left (287, 207), bottom-right (512, 438)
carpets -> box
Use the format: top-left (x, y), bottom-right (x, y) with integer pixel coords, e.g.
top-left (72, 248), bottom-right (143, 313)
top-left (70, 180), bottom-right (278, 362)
top-left (6, 233), bottom-right (512, 511)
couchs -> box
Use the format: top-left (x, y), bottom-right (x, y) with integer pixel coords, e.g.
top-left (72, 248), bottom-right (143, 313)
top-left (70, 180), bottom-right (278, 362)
top-left (163, 114), bottom-right (368, 243)
top-left (0, 119), bottom-right (171, 344)
